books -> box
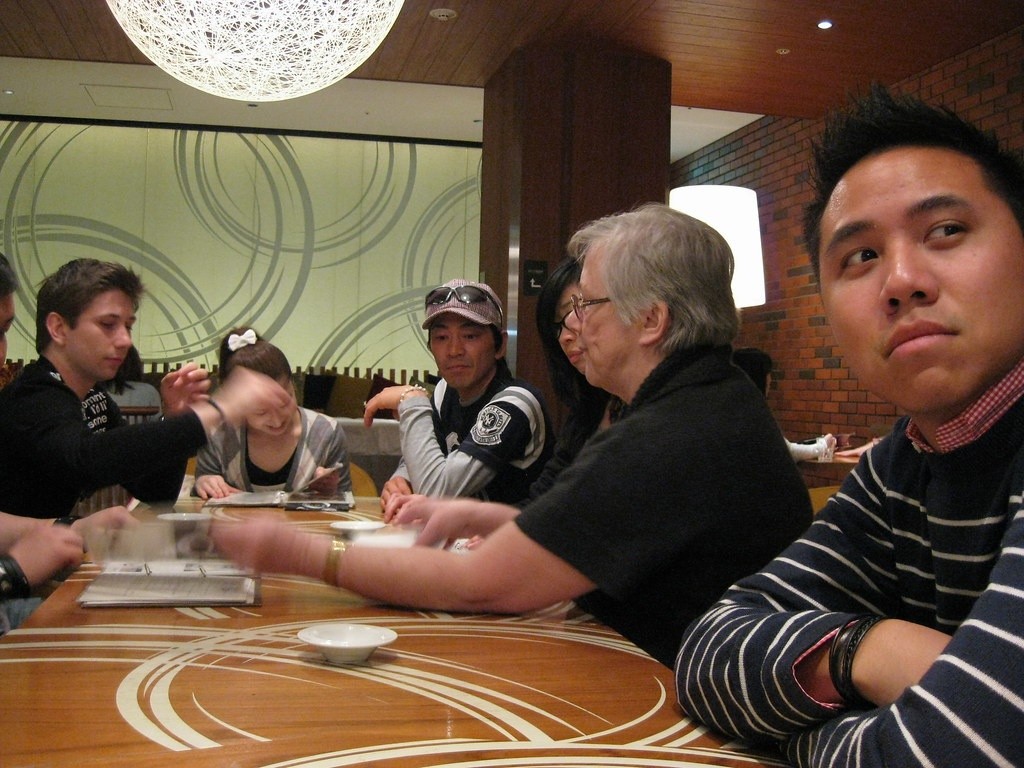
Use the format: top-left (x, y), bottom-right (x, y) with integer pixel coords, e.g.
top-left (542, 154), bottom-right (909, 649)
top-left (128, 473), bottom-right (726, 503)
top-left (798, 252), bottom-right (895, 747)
top-left (78, 559), bottom-right (260, 609)
top-left (201, 489), bottom-right (356, 512)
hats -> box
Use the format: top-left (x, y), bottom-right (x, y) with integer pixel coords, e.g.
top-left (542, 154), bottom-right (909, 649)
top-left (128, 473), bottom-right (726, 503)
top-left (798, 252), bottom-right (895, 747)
top-left (422, 279), bottom-right (505, 331)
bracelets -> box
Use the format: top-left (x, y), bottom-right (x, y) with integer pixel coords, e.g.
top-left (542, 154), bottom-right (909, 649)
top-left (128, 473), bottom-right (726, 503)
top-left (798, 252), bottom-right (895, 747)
top-left (54, 515), bottom-right (82, 527)
top-left (322, 540), bottom-right (348, 588)
top-left (399, 385), bottom-right (428, 403)
top-left (205, 396), bottom-right (226, 425)
top-left (0, 553), bottom-right (29, 598)
top-left (829, 612), bottom-right (886, 712)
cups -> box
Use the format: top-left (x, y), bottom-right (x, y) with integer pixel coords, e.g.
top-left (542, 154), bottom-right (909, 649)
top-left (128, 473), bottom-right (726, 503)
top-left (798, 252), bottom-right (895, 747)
top-left (816, 437), bottom-right (835, 463)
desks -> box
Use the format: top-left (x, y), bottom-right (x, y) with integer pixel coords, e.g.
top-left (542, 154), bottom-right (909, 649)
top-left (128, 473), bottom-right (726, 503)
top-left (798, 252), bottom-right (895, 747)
top-left (797, 454), bottom-right (861, 471)
top-left (0, 503), bottom-right (793, 767)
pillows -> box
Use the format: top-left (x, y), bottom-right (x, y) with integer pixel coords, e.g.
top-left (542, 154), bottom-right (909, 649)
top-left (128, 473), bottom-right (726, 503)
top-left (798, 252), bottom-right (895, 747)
top-left (324, 370), bottom-right (373, 418)
top-left (411, 377), bottom-right (435, 399)
top-left (293, 371), bottom-right (306, 408)
top-left (303, 374), bottom-right (337, 413)
top-left (424, 374), bottom-right (441, 385)
top-left (363, 374), bottom-right (405, 419)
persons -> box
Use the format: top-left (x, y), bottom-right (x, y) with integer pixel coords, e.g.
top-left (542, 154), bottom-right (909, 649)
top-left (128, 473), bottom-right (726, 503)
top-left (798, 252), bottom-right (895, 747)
top-left (363, 257), bottom-right (627, 551)
top-left (184, 327), bottom-right (354, 500)
top-left (732, 345), bottom-right (833, 464)
top-left (675, 79), bottom-right (1024, 768)
top-left (211, 201), bottom-right (813, 671)
top-left (0, 252), bottom-right (289, 637)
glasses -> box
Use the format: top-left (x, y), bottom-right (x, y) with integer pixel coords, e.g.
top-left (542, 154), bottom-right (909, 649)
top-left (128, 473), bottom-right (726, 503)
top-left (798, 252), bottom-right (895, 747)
top-left (571, 292), bottom-right (616, 321)
top-left (546, 308), bottom-right (574, 339)
top-left (424, 286), bottom-right (503, 326)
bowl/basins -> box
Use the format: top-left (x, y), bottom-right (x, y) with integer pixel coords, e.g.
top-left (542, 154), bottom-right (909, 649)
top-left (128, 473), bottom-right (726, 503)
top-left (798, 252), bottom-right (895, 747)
top-left (157, 512), bottom-right (210, 538)
top-left (330, 520), bottom-right (387, 540)
top-left (297, 623), bottom-right (398, 664)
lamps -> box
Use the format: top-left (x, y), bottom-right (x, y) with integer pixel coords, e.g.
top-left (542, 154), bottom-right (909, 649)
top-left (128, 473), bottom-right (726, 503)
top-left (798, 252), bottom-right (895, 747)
top-left (669, 185), bottom-right (767, 307)
top-left (105, 0), bottom-right (405, 104)
